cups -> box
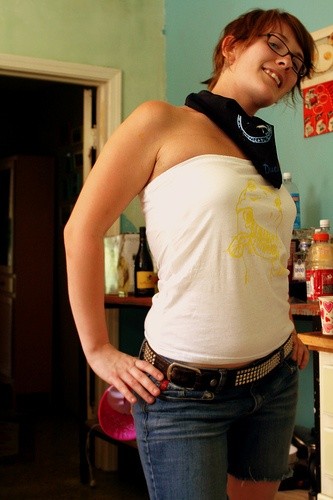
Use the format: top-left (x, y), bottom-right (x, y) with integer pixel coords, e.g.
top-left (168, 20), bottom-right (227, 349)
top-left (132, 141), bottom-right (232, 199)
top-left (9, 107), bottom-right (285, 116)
top-left (318, 296), bottom-right (333, 335)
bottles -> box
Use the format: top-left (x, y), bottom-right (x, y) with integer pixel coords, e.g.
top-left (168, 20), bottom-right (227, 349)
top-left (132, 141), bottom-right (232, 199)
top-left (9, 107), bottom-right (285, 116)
top-left (311, 229), bottom-right (321, 244)
top-left (134, 226), bottom-right (155, 298)
top-left (282, 172), bottom-right (301, 237)
top-left (292, 242), bottom-right (309, 280)
top-left (305, 233), bottom-right (333, 305)
top-left (319, 220), bottom-right (333, 244)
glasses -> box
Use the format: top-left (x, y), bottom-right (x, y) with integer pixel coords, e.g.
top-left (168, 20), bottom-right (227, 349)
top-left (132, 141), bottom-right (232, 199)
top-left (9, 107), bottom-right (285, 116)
top-left (255, 31), bottom-right (309, 77)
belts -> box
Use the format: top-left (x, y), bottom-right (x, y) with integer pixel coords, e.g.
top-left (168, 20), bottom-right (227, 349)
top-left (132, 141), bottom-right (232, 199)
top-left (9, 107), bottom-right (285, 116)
top-left (141, 334), bottom-right (294, 391)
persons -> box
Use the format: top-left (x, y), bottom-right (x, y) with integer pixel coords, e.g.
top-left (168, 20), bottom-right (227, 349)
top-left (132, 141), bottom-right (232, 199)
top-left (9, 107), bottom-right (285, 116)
top-left (64, 7), bottom-right (317, 500)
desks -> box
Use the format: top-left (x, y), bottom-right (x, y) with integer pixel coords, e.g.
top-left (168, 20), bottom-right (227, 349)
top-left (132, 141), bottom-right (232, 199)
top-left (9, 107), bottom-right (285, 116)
top-left (99, 293), bottom-right (326, 500)
